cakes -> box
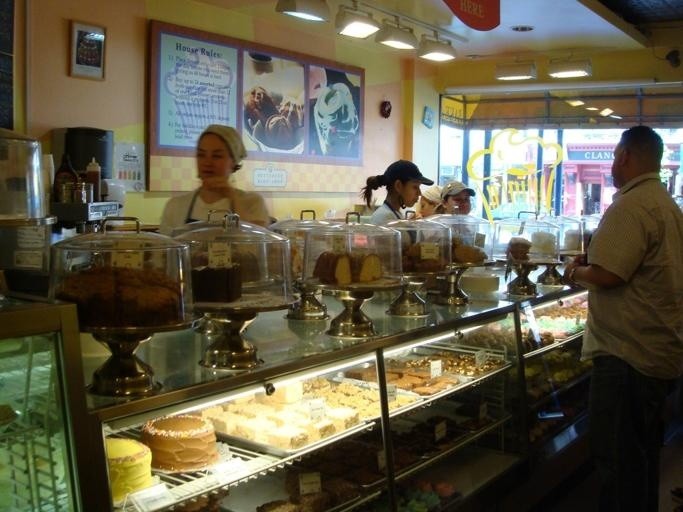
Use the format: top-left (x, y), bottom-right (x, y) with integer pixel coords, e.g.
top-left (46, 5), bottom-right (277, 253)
top-left (506, 236), bottom-right (530, 260)
top-left (346, 361), bottom-right (456, 397)
top-left (450, 238), bottom-right (490, 263)
top-left (104, 437), bottom-right (151, 502)
top-left (193, 262), bottom-right (241, 301)
top-left (200, 390), bottom-right (358, 452)
top-left (58, 264), bottom-right (180, 323)
top-left (531, 232), bottom-right (557, 255)
top-left (311, 249), bottom-right (384, 285)
top-left (143, 414), bottom-right (221, 469)
top-left (310, 378), bottom-right (415, 422)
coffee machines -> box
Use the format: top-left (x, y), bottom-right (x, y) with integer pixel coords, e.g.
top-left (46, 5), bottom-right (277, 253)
top-left (47, 126), bottom-right (126, 231)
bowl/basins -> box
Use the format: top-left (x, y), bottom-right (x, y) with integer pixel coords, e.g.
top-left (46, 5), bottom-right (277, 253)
top-left (246, 52), bottom-right (272, 64)
top-left (242, 108), bottom-right (305, 154)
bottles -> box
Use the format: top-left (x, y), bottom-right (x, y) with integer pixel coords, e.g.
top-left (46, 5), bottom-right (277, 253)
top-left (54, 154), bottom-right (101, 208)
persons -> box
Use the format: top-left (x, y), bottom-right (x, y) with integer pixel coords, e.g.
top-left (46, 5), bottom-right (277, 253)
top-left (441, 181), bottom-right (475, 215)
top-left (417, 185), bottom-right (444, 217)
top-left (160, 123), bottom-right (272, 236)
top-left (357, 160), bottom-right (435, 256)
top-left (563, 124), bottom-right (683, 512)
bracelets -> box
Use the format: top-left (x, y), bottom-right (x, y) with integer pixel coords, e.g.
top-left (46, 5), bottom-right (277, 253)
top-left (567, 266), bottom-right (584, 285)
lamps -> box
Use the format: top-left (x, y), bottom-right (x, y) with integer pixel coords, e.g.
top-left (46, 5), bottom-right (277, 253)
top-left (275, 0), bottom-right (469, 63)
top-left (472, 46), bottom-right (611, 80)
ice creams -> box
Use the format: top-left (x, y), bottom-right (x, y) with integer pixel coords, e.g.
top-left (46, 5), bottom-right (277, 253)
top-left (314, 80), bottom-right (359, 157)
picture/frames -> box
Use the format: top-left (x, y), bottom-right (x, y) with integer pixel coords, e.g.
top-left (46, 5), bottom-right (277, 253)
top-left (142, 18), bottom-right (365, 167)
top-left (69, 18), bottom-right (108, 81)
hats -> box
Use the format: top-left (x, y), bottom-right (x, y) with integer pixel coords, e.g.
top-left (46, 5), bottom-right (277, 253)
top-left (385, 160), bottom-right (433, 186)
top-left (441, 181), bottom-right (475, 204)
